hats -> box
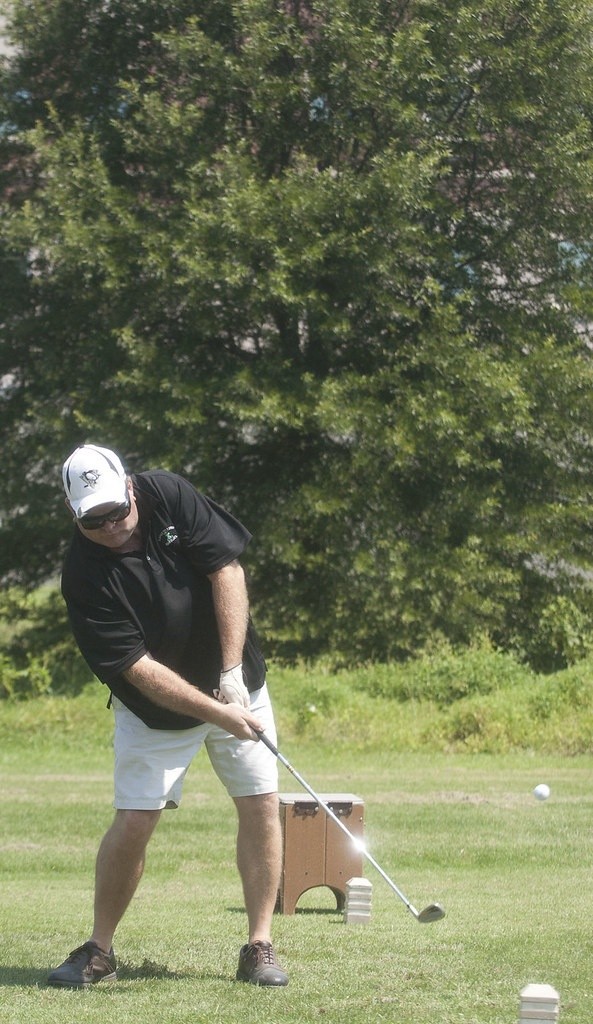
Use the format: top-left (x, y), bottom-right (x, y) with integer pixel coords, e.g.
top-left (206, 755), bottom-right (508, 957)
top-left (61, 445), bottom-right (129, 520)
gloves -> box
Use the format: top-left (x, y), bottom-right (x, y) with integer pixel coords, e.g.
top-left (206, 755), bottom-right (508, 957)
top-left (213, 668), bottom-right (250, 713)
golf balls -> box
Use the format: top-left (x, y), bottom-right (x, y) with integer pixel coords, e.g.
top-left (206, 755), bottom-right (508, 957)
top-left (534, 784), bottom-right (550, 800)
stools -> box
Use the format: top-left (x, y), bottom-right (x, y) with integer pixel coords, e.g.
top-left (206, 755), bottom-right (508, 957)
top-left (278, 793), bottom-right (364, 914)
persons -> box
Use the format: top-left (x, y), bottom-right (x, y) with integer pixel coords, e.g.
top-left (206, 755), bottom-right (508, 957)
top-left (49, 444), bottom-right (290, 990)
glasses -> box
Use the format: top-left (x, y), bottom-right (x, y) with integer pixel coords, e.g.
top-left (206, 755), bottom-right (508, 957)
top-left (79, 487), bottom-right (132, 530)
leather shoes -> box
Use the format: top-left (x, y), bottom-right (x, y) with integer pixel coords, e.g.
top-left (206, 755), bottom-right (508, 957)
top-left (236, 945), bottom-right (288, 989)
top-left (47, 942), bottom-right (118, 988)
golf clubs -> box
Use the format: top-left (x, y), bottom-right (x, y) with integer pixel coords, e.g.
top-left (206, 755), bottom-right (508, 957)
top-left (253, 728), bottom-right (446, 924)
